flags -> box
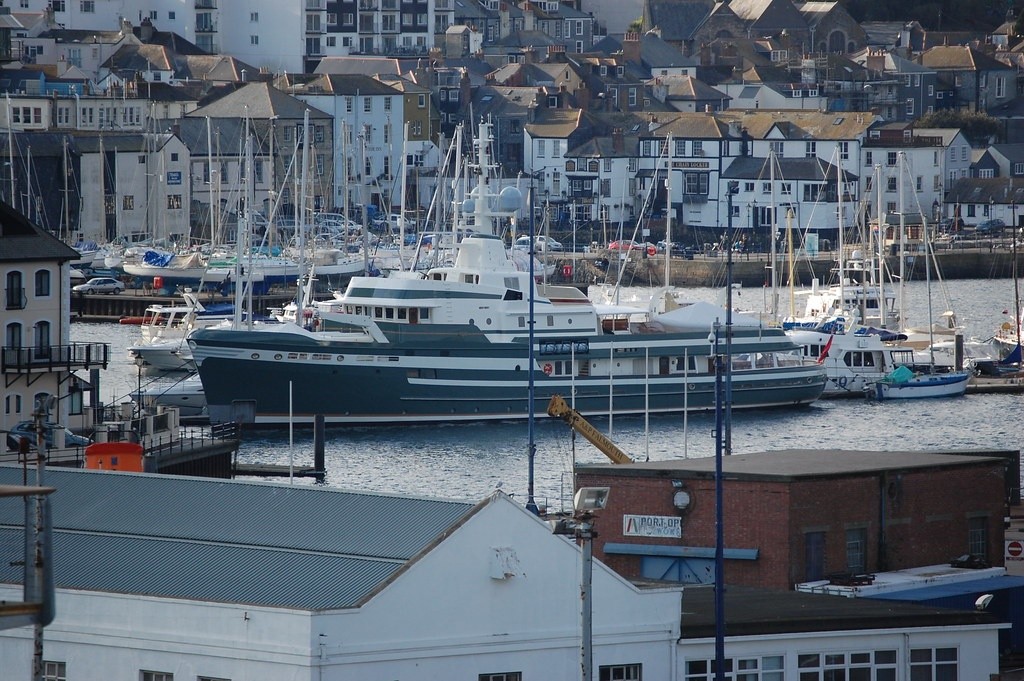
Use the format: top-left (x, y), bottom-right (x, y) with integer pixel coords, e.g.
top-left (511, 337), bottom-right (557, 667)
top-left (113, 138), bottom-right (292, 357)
top-left (818, 335), bottom-right (833, 363)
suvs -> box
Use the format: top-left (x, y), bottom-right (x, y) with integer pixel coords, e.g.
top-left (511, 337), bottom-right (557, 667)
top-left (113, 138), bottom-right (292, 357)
top-left (6, 421), bottom-right (94, 452)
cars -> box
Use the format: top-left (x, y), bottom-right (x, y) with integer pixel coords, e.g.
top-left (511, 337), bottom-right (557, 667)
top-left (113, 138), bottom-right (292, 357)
top-left (275, 220), bottom-right (306, 236)
top-left (608, 240), bottom-right (645, 253)
top-left (744, 235), bottom-right (780, 253)
top-left (318, 220), bottom-right (344, 234)
top-left (73, 278), bottom-right (124, 295)
top-left (535, 236), bottom-right (564, 252)
top-left (937, 218), bottom-right (964, 233)
top-left (372, 214), bottom-right (409, 232)
top-left (516, 236), bottom-right (538, 254)
top-left (339, 221), bottom-right (363, 236)
top-left (251, 213), bottom-right (269, 233)
top-left (656, 241), bottom-right (683, 255)
top-left (281, 203), bottom-right (312, 217)
top-left (974, 219), bottom-right (1005, 234)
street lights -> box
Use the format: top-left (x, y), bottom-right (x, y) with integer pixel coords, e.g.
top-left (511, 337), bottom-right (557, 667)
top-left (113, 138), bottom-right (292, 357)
top-left (988, 193), bottom-right (994, 253)
top-left (135, 349), bottom-right (144, 436)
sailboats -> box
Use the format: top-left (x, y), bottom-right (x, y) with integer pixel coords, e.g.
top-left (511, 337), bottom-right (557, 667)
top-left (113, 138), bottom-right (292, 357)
top-left (0, 91), bottom-right (1024, 425)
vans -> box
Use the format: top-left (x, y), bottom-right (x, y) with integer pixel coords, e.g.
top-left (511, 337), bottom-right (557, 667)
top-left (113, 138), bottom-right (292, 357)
top-left (313, 212), bottom-right (349, 220)
